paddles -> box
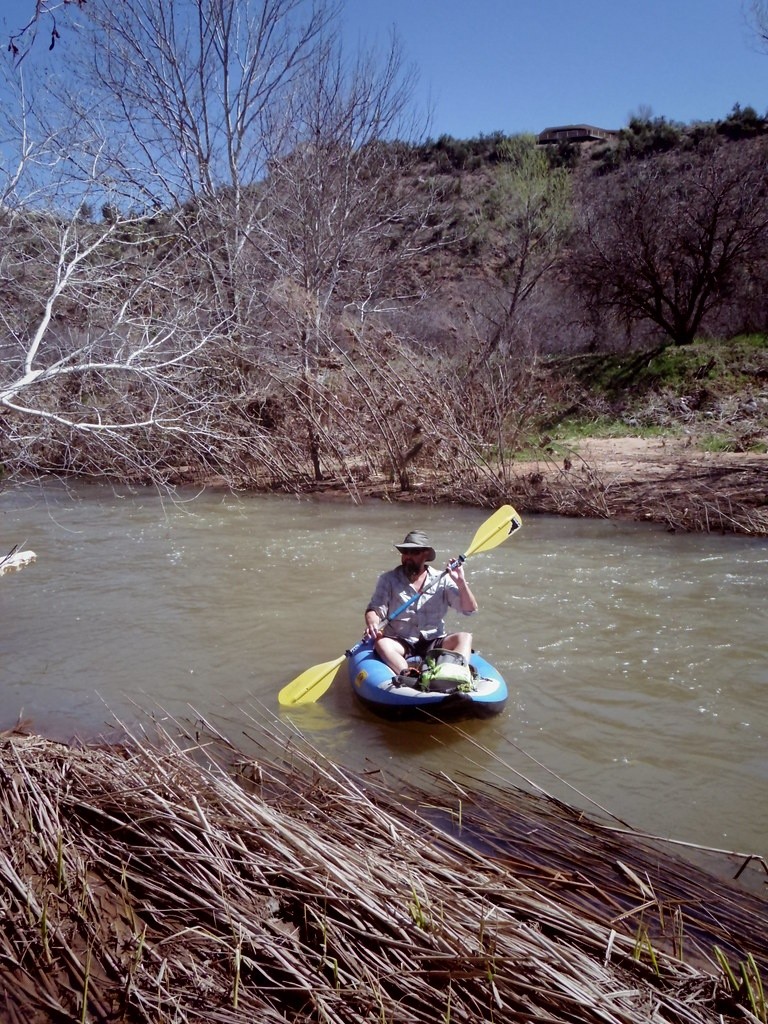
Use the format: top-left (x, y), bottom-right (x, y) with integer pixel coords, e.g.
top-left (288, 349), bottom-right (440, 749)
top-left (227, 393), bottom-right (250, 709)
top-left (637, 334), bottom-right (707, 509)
top-left (276, 503), bottom-right (524, 709)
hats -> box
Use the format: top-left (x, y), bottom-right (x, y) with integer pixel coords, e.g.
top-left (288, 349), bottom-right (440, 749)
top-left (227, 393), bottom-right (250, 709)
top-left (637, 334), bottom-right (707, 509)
top-left (393, 529), bottom-right (436, 562)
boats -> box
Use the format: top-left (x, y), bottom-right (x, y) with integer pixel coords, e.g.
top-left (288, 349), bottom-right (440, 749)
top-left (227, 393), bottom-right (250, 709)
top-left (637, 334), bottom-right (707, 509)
top-left (347, 635), bottom-right (510, 718)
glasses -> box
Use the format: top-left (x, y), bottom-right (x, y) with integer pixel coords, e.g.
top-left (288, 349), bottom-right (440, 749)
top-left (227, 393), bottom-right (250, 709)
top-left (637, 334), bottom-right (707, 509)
top-left (397, 546), bottom-right (428, 555)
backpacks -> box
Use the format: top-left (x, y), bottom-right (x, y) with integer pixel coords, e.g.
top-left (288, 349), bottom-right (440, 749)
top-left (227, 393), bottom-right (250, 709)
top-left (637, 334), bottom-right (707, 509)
top-left (421, 647), bottom-right (475, 692)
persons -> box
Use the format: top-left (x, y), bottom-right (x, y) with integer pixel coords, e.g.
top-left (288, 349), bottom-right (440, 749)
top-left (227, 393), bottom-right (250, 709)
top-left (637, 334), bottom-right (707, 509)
top-left (362, 528), bottom-right (480, 690)
